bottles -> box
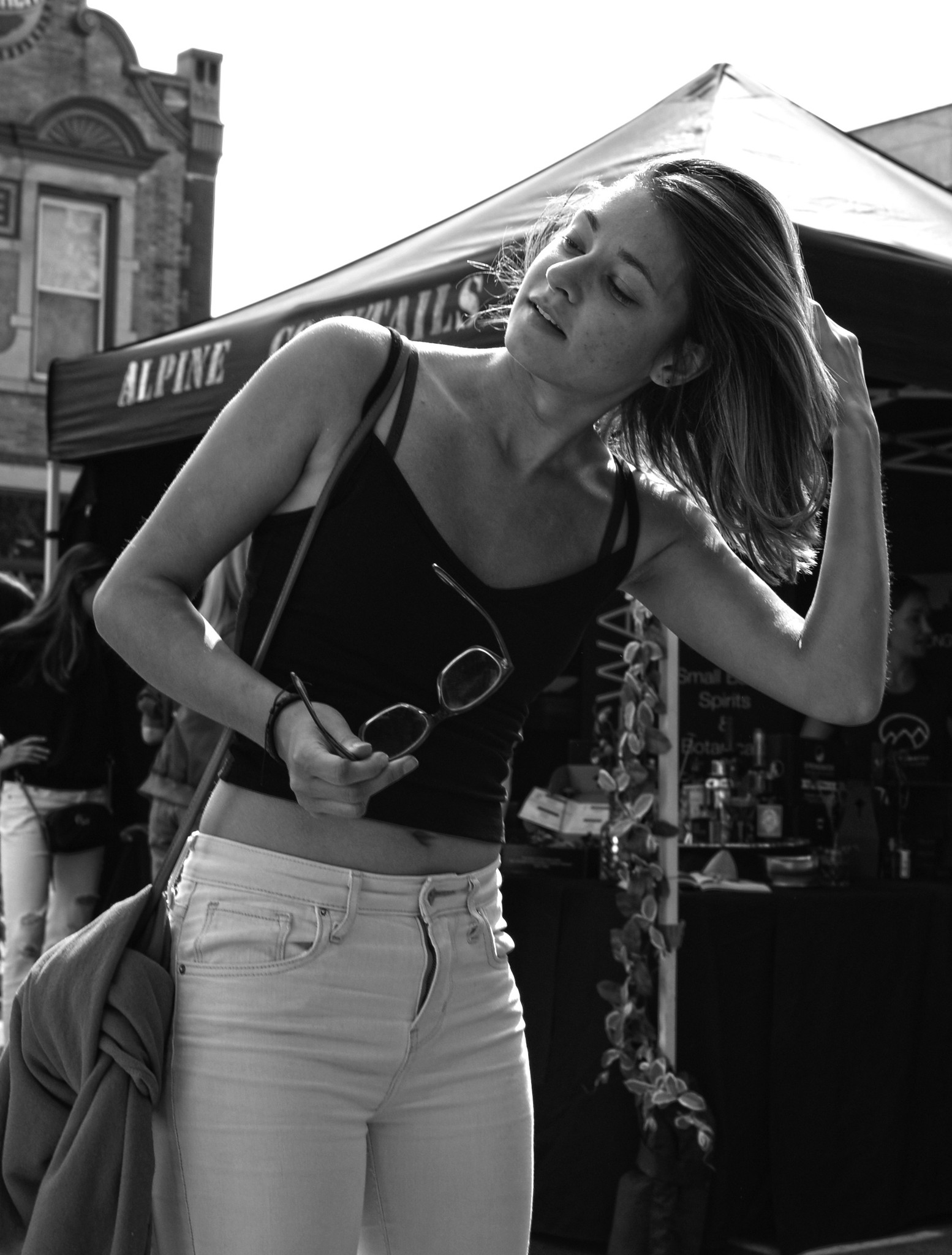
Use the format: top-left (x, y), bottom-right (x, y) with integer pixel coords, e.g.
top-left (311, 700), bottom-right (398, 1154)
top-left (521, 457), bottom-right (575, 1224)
top-left (745, 726), bottom-right (768, 793)
top-left (704, 756), bottom-right (731, 845)
top-left (755, 763), bottom-right (788, 842)
top-left (680, 757), bottom-right (713, 844)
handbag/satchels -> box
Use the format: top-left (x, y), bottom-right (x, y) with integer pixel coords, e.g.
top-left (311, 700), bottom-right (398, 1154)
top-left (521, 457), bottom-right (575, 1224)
top-left (41, 803), bottom-right (116, 853)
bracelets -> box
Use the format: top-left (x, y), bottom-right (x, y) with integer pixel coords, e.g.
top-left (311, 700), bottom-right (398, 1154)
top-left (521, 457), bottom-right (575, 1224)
top-left (264, 681), bottom-right (312, 764)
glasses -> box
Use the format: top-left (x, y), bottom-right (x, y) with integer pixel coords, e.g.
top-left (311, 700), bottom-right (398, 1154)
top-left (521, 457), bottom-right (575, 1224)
top-left (291, 563), bottom-right (513, 763)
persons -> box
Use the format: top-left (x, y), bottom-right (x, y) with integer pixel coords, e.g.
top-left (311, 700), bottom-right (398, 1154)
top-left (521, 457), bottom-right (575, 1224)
top-left (3, 155), bottom-right (932, 1255)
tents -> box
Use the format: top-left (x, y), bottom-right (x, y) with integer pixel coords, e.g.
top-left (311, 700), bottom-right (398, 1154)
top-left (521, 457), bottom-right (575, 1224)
top-left (46, 64), bottom-right (952, 1073)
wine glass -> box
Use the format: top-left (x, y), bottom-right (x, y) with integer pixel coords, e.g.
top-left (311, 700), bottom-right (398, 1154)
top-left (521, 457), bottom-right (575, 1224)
top-left (722, 798), bottom-right (756, 844)
